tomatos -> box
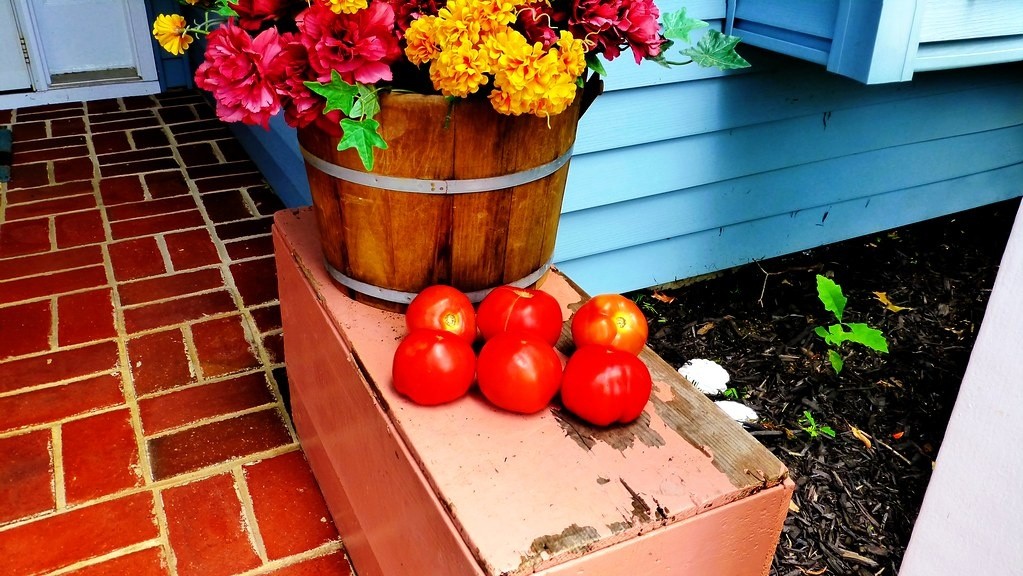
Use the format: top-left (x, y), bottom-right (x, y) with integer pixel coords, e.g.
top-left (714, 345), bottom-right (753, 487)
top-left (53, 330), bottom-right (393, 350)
top-left (391, 284), bottom-right (652, 427)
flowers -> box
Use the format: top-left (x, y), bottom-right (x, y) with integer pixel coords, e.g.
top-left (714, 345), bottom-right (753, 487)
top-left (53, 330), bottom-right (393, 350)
top-left (149, 0), bottom-right (754, 171)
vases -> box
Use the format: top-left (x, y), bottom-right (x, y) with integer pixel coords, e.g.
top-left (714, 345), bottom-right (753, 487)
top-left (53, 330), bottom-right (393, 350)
top-left (297, 92), bottom-right (583, 314)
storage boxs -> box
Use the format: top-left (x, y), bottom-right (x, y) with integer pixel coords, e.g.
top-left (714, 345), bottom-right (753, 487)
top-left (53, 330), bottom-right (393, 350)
top-left (266, 204), bottom-right (799, 576)
